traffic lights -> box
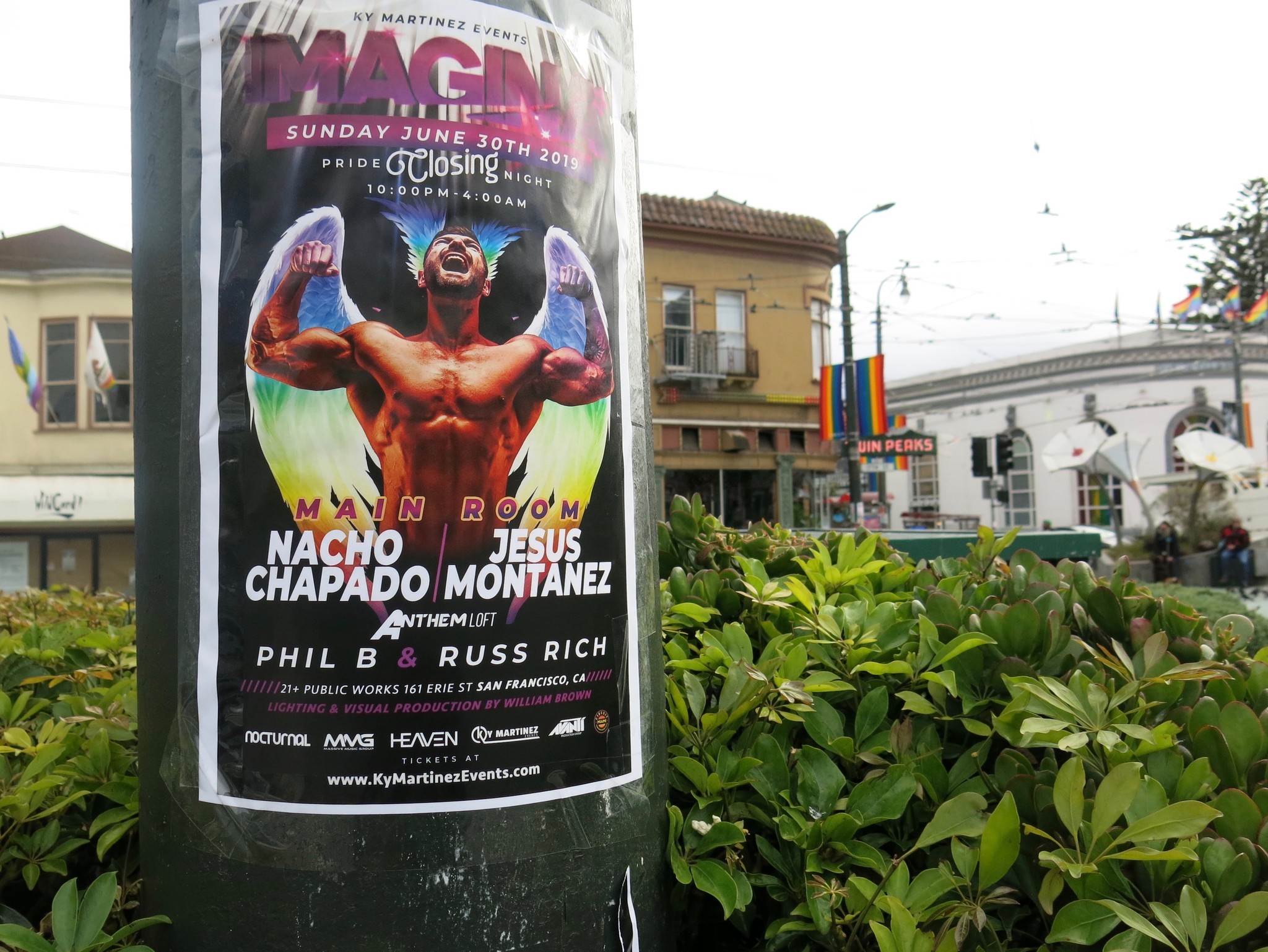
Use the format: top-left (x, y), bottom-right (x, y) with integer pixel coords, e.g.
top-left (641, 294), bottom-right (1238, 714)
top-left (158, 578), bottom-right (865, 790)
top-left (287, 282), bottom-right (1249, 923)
top-left (971, 437), bottom-right (986, 476)
top-left (994, 434), bottom-right (1015, 475)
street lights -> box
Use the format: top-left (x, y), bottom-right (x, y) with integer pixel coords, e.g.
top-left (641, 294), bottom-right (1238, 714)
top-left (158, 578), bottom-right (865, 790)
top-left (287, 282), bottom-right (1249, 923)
top-left (838, 201), bottom-right (897, 524)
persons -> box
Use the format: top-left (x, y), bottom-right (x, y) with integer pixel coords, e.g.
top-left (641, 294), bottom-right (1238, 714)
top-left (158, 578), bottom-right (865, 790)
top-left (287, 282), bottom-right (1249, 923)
top-left (1150, 521), bottom-right (1180, 583)
top-left (1217, 518), bottom-right (1250, 586)
top-left (245, 226), bottom-right (616, 622)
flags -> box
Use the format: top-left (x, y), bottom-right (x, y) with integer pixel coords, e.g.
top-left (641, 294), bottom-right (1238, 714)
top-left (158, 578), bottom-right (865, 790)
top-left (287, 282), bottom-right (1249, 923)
top-left (83, 321), bottom-right (115, 407)
top-left (8, 327), bottom-right (43, 414)
top-left (1242, 291), bottom-right (1268, 326)
top-left (1219, 284), bottom-right (1240, 322)
top-left (818, 363), bottom-right (848, 442)
top-left (853, 354), bottom-right (889, 437)
top-left (1172, 287), bottom-right (1204, 325)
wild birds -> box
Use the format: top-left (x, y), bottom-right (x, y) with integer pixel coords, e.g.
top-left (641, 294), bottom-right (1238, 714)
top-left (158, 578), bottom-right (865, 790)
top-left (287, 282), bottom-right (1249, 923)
top-left (1037, 203), bottom-right (1059, 216)
top-left (894, 260), bottom-right (921, 274)
top-left (1049, 242), bottom-right (1081, 265)
